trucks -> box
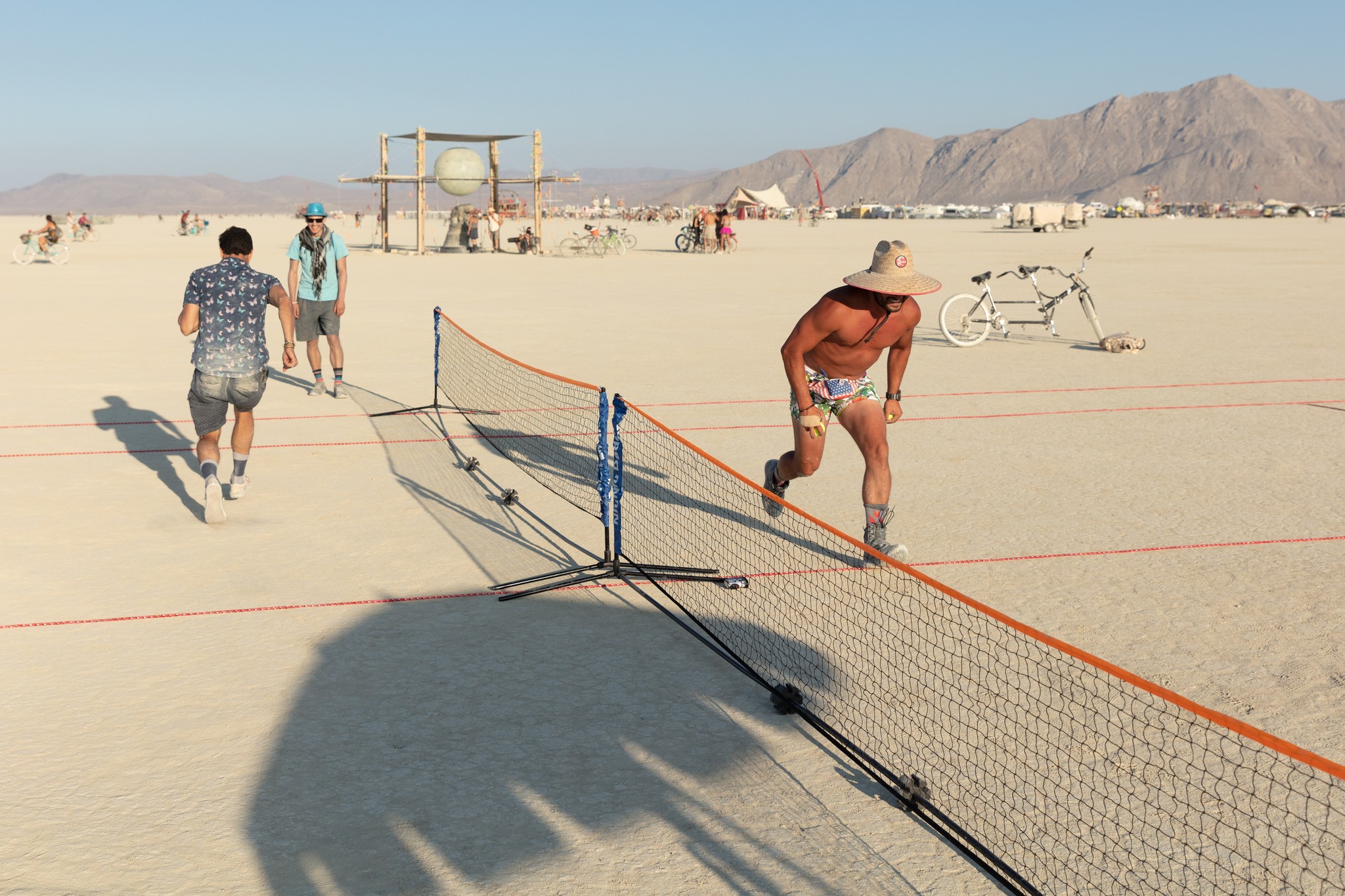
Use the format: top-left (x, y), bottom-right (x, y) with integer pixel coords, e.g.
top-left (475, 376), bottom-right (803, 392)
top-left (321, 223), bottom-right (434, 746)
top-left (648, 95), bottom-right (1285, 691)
top-left (1011, 202), bottom-right (1086, 233)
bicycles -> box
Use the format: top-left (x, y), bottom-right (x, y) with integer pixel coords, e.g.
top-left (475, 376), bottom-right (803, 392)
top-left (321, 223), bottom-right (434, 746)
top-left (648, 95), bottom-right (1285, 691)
top-left (939, 246), bottom-right (1104, 348)
top-left (519, 233), bottom-right (537, 255)
top-left (558, 220), bottom-right (638, 258)
top-left (176, 218), bottom-right (209, 237)
top-left (675, 222), bottom-right (738, 254)
top-left (12, 230), bottom-right (70, 265)
top-left (60, 222), bottom-right (101, 244)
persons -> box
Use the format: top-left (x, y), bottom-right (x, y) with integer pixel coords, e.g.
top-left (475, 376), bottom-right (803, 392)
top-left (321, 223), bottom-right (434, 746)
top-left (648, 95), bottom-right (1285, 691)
top-left (179, 225), bottom-right (299, 525)
top-left (287, 203), bottom-right (349, 398)
top-left (691, 202), bottom-right (734, 255)
top-left (31, 210), bottom-right (94, 259)
top-left (762, 240), bottom-right (941, 565)
top-left (352, 207), bottom-right (540, 254)
top-left (178, 209), bottom-right (208, 236)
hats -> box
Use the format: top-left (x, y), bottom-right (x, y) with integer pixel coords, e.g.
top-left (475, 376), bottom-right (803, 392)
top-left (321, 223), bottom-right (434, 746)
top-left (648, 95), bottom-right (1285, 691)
top-left (695, 210), bottom-right (702, 217)
top-left (487, 207), bottom-right (496, 212)
top-left (707, 205), bottom-right (714, 210)
top-left (302, 203), bottom-right (329, 218)
top-left (842, 239), bottom-right (942, 295)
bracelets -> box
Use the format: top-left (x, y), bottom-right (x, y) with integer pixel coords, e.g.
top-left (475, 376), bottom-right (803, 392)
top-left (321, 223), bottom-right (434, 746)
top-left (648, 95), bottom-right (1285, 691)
top-left (283, 341), bottom-right (295, 350)
top-left (292, 300), bottom-right (299, 306)
top-left (798, 403), bottom-right (814, 412)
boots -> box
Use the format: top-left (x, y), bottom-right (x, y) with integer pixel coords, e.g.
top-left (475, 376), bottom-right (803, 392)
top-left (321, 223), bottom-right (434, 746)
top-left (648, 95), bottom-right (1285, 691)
top-left (762, 459), bottom-right (789, 517)
top-left (862, 505), bottom-right (908, 568)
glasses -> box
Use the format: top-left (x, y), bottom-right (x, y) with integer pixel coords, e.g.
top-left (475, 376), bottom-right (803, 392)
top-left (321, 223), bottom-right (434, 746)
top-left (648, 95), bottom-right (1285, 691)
top-left (306, 216), bottom-right (324, 223)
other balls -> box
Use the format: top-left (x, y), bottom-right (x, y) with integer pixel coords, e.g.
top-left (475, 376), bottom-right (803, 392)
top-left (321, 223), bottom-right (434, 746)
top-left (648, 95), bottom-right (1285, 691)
top-left (812, 422), bottom-right (825, 435)
top-left (882, 409), bottom-right (894, 419)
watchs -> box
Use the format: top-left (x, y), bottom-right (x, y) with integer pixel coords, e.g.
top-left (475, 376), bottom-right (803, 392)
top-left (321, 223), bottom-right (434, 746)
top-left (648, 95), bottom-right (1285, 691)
top-left (885, 389), bottom-right (903, 402)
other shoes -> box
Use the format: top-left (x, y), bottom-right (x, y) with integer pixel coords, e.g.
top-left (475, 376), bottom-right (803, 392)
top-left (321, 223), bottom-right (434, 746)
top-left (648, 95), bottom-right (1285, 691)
top-left (493, 248), bottom-right (498, 253)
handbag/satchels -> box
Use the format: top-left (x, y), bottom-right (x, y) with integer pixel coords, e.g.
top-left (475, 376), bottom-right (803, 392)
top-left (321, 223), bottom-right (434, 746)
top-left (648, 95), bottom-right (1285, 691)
top-left (55, 228), bottom-right (63, 238)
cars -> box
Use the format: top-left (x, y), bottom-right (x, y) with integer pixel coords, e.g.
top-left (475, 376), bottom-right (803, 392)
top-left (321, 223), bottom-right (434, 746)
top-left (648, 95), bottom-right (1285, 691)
top-left (1084, 197), bottom-right (1345, 218)
top-left (541, 202), bottom-right (1014, 223)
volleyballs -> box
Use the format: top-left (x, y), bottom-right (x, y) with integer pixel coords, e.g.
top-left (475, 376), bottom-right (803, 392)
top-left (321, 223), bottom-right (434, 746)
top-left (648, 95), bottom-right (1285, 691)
top-left (435, 148), bottom-right (484, 195)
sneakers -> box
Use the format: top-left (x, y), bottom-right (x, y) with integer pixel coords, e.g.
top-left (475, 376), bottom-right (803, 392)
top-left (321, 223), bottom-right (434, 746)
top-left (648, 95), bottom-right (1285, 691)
top-left (309, 381), bottom-right (327, 396)
top-left (205, 478), bottom-right (226, 524)
top-left (230, 475), bottom-right (250, 498)
top-left (334, 383), bottom-right (348, 398)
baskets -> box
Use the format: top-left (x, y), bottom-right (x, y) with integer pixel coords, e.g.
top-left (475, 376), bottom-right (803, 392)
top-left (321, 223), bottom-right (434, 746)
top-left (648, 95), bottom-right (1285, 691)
top-left (20, 235), bottom-right (29, 244)
